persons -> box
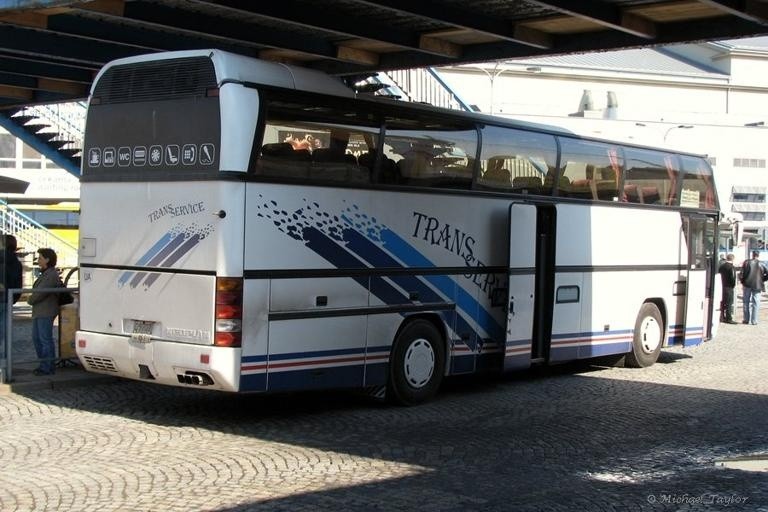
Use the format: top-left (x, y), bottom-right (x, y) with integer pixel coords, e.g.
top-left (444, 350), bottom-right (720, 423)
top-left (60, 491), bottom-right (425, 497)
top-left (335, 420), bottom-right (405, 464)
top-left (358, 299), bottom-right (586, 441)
top-left (0, 234), bottom-right (23, 358)
top-left (755, 239), bottom-right (768, 249)
top-left (719, 253), bottom-right (726, 260)
top-left (27, 248), bottom-right (74, 375)
top-left (720, 254), bottom-right (738, 324)
top-left (739, 250), bottom-right (768, 324)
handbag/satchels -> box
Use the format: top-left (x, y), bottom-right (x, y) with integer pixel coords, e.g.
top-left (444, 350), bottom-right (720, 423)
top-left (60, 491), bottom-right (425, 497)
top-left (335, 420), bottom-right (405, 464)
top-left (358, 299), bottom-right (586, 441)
top-left (54, 278), bottom-right (75, 305)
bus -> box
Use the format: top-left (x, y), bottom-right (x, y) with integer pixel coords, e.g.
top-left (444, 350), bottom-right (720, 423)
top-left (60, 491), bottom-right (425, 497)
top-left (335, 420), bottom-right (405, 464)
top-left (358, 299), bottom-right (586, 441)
top-left (703, 208), bottom-right (767, 272)
top-left (0, 200), bottom-right (80, 295)
top-left (69, 46), bottom-right (743, 416)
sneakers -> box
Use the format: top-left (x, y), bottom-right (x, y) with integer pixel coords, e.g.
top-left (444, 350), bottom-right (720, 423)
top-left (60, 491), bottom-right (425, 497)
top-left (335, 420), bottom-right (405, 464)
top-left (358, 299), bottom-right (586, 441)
top-left (32, 367), bottom-right (52, 375)
top-left (720, 316), bottom-right (757, 325)
